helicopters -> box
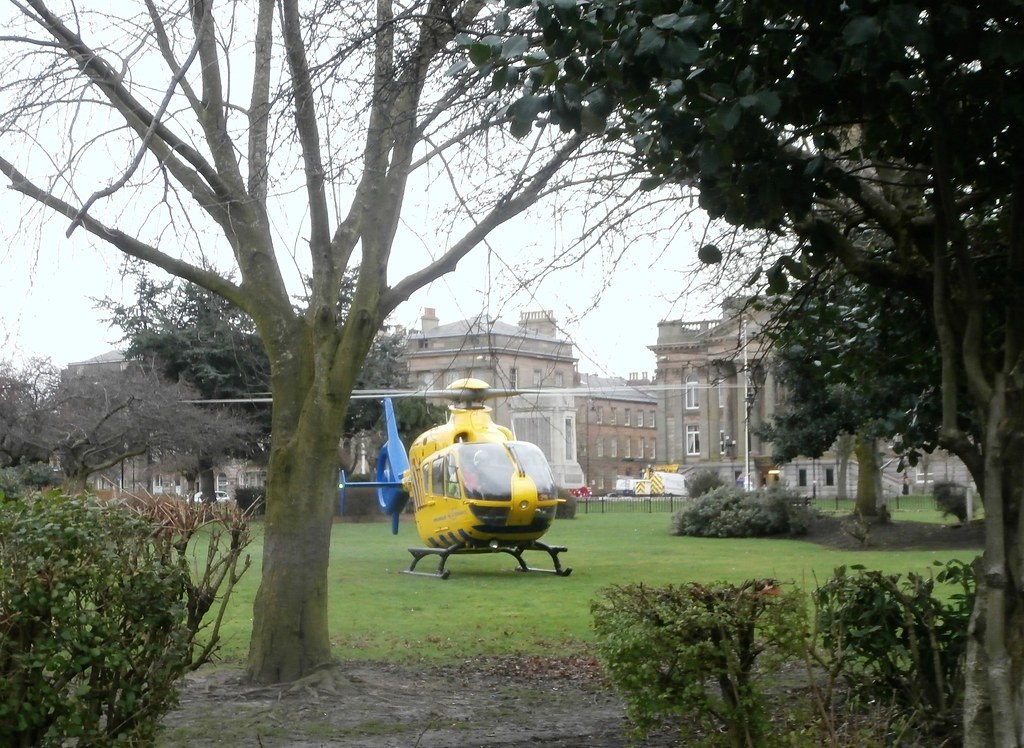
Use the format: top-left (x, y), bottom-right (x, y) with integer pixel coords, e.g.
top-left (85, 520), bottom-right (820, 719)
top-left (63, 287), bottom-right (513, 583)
top-left (183, 377), bottom-right (768, 579)
